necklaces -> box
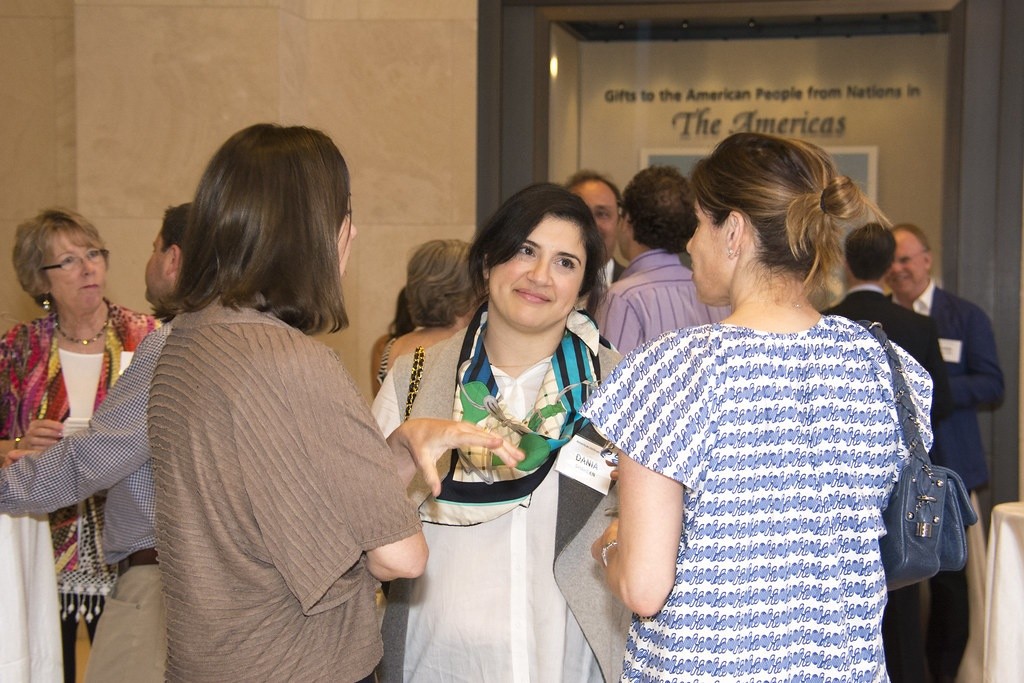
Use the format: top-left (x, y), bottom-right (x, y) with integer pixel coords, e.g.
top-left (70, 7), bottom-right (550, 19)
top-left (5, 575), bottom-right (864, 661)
top-left (56, 312), bottom-right (110, 345)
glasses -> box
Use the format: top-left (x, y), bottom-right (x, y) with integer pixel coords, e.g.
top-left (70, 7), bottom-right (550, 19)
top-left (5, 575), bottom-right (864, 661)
top-left (892, 250), bottom-right (926, 265)
top-left (39, 248), bottom-right (110, 272)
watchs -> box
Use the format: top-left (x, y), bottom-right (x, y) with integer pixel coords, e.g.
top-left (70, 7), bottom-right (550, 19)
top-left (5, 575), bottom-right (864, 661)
top-left (599, 539), bottom-right (618, 567)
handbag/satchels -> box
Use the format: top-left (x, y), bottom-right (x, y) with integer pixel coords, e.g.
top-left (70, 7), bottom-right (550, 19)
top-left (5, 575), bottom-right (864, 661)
top-left (853, 319), bottom-right (978, 591)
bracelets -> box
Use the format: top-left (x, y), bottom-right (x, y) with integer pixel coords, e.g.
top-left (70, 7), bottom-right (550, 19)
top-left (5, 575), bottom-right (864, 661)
top-left (14, 437), bottom-right (21, 449)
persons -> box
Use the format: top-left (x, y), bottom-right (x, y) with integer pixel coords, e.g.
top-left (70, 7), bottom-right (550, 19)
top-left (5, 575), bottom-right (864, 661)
top-left (148, 124), bottom-right (1005, 683)
top-left (0, 202), bottom-right (189, 683)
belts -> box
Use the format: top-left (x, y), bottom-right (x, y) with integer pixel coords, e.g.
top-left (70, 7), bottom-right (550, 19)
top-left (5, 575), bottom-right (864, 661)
top-left (117, 548), bottom-right (158, 576)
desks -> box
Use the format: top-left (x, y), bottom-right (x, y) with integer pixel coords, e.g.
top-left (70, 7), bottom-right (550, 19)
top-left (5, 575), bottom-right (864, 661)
top-left (982, 500), bottom-right (1024, 683)
